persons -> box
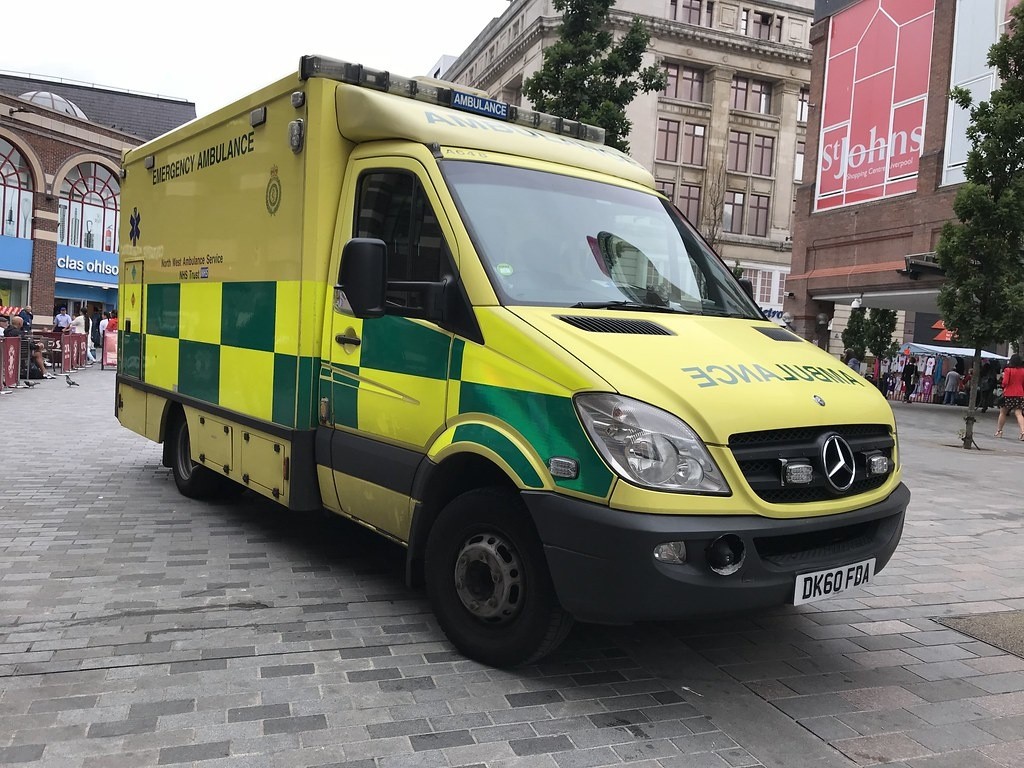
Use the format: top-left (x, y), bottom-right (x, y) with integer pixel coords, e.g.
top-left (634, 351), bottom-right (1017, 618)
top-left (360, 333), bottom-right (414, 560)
top-left (844, 349), bottom-right (1024, 441)
top-left (0, 306), bottom-right (118, 380)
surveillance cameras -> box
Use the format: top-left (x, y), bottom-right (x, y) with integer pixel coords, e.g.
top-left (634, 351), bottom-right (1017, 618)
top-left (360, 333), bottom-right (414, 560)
top-left (784, 292), bottom-right (794, 297)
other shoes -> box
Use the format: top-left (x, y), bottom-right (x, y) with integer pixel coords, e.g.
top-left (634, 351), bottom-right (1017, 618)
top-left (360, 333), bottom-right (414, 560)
top-left (902, 396), bottom-right (905, 402)
top-left (907, 400), bottom-right (912, 403)
top-left (87, 359), bottom-right (96, 365)
top-left (43, 373), bottom-right (55, 379)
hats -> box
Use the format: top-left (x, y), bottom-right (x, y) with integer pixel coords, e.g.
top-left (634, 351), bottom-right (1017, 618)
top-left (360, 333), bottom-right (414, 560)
top-left (61, 308), bottom-right (66, 311)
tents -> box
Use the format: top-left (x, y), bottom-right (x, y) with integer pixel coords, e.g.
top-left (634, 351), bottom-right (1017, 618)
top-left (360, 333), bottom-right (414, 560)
top-left (897, 342), bottom-right (1009, 361)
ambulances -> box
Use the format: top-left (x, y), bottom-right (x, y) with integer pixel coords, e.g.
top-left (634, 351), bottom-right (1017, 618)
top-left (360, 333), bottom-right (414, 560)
top-left (112, 54), bottom-right (912, 676)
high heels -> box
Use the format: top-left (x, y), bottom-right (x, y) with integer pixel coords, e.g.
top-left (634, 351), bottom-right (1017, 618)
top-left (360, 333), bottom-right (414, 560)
top-left (995, 430), bottom-right (1002, 438)
top-left (1019, 432), bottom-right (1024, 440)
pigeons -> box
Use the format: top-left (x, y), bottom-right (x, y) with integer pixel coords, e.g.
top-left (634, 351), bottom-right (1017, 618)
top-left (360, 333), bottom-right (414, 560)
top-left (64, 375), bottom-right (80, 389)
top-left (24, 380), bottom-right (41, 389)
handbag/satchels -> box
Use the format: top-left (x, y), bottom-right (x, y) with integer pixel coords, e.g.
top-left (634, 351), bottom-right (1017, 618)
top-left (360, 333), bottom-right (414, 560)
top-left (983, 378), bottom-right (994, 391)
top-left (911, 366), bottom-right (918, 384)
top-left (993, 394), bottom-right (1004, 406)
